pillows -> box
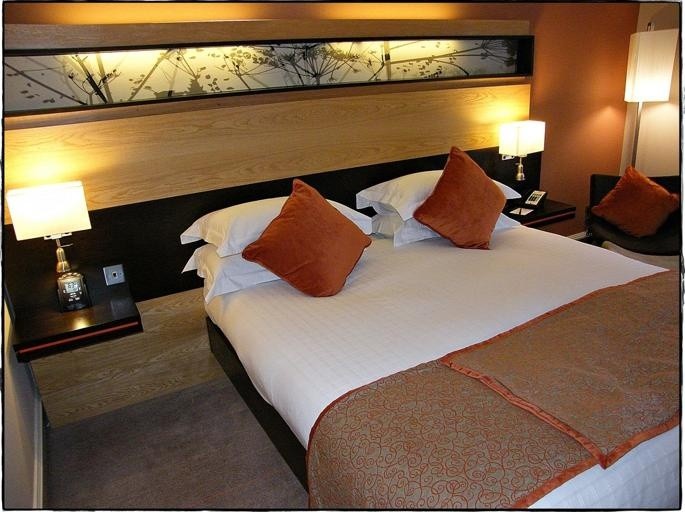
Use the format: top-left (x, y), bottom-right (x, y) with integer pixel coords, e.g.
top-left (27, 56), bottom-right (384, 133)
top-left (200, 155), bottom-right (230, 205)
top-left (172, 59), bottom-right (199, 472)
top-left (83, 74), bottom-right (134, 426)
top-left (178, 191), bottom-right (377, 257)
top-left (594, 169), bottom-right (678, 241)
top-left (353, 171), bottom-right (528, 219)
top-left (365, 209), bottom-right (523, 248)
top-left (179, 244), bottom-right (358, 299)
top-left (413, 141), bottom-right (515, 253)
top-left (240, 176), bottom-right (374, 301)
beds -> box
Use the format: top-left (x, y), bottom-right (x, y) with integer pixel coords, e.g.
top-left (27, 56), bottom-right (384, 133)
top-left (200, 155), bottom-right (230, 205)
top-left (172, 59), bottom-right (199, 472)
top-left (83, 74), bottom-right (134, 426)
top-left (200, 212), bottom-right (680, 510)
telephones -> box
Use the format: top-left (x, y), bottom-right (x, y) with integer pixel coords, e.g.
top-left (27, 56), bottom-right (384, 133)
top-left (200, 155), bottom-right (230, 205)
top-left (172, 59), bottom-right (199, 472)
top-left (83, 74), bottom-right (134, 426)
top-left (520, 187), bottom-right (548, 207)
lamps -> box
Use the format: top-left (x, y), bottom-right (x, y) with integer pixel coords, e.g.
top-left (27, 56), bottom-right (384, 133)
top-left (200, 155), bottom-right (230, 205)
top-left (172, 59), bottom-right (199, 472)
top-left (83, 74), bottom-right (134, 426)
top-left (620, 20), bottom-right (681, 167)
top-left (496, 118), bottom-right (548, 181)
top-left (5, 178), bottom-right (95, 275)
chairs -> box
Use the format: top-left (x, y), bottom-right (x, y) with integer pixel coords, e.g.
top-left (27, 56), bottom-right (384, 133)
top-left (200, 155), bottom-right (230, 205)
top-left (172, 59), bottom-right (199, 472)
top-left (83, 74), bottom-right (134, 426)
top-left (581, 171), bottom-right (681, 269)
